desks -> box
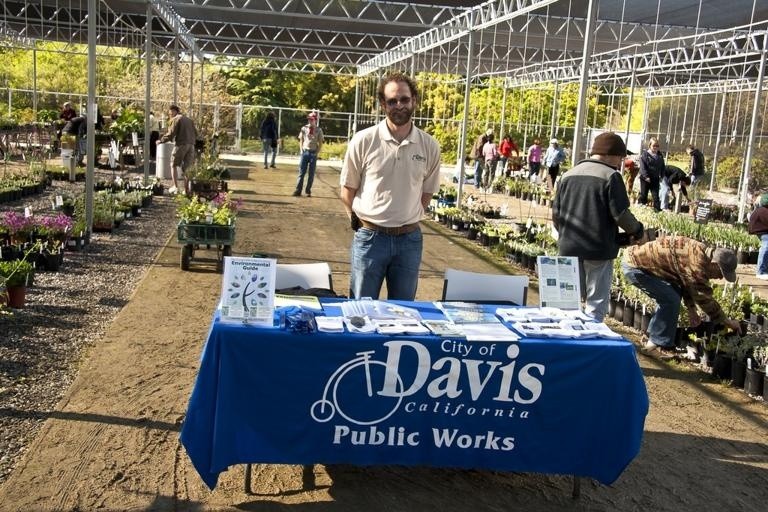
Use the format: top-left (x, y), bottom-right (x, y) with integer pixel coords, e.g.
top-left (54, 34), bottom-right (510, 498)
top-left (212, 296), bottom-right (633, 499)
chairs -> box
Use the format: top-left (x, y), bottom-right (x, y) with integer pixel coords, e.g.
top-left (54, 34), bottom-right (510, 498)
top-left (273, 262), bottom-right (342, 295)
top-left (436, 266), bottom-right (529, 307)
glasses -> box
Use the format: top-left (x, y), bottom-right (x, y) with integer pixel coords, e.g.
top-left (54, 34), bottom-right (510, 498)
top-left (652, 145), bottom-right (659, 147)
top-left (387, 97), bottom-right (410, 106)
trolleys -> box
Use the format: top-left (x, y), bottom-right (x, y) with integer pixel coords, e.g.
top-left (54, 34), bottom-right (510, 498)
top-left (176, 178), bottom-right (236, 271)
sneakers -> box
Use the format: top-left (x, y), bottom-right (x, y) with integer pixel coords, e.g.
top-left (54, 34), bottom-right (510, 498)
top-left (756, 274), bottom-right (768, 281)
top-left (169, 164), bottom-right (310, 198)
top-left (641, 346), bottom-right (675, 358)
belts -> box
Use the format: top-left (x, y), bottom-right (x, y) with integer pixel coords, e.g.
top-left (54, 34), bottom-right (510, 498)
top-left (359, 219), bottom-right (419, 234)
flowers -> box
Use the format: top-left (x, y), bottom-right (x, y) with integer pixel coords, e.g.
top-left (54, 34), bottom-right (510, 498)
top-left (174, 189), bottom-right (243, 227)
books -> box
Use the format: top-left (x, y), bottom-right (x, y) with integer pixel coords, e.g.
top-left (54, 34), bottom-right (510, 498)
top-left (273, 293), bottom-right (624, 342)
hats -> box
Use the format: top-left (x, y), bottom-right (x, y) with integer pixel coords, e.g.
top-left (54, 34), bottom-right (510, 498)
top-left (306, 113), bottom-right (317, 120)
top-left (549, 138), bottom-right (558, 144)
top-left (591, 132), bottom-right (626, 156)
top-left (711, 248), bottom-right (737, 282)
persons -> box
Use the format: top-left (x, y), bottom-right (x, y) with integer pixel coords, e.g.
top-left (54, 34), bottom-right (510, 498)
top-left (57, 100), bottom-right (119, 169)
top-left (621, 234), bottom-right (743, 363)
top-left (259, 112), bottom-right (280, 170)
top-left (746, 192), bottom-right (768, 281)
top-left (550, 131), bottom-right (647, 326)
top-left (292, 112), bottom-right (325, 199)
top-left (338, 74), bottom-right (442, 302)
top-left (468, 128), bottom-right (708, 214)
top-left (154, 105), bottom-right (198, 196)
